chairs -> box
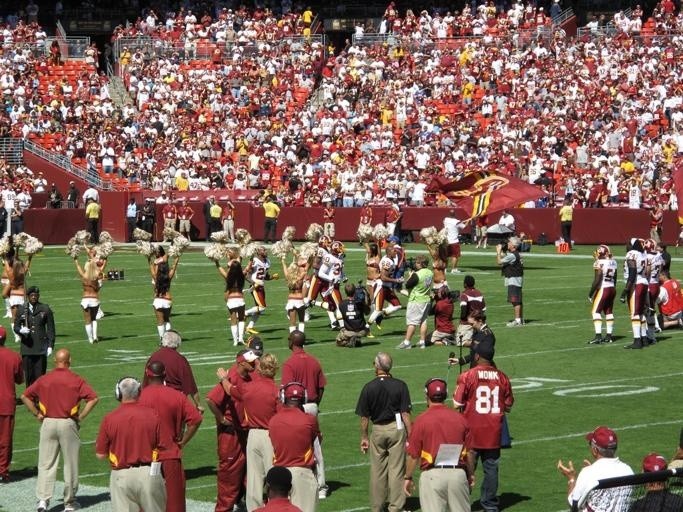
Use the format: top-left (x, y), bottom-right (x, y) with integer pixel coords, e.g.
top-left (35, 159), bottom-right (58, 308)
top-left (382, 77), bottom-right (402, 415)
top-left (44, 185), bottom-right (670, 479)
top-left (27, 58), bottom-right (143, 194)
top-left (181, 57), bottom-right (311, 129)
top-left (392, 98), bottom-right (489, 151)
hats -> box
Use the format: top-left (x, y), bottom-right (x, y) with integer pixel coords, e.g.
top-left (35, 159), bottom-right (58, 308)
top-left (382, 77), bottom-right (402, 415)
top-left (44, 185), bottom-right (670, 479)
top-left (26, 286), bottom-right (38, 296)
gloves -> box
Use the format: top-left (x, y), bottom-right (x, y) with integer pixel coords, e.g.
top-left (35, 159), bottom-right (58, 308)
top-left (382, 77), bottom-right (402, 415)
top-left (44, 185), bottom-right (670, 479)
top-left (47, 347), bottom-right (52, 357)
top-left (20, 327), bottom-right (30, 335)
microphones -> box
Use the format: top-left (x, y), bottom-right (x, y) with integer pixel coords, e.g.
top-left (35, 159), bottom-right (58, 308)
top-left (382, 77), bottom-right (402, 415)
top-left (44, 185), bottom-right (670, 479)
top-left (448, 352), bottom-right (455, 369)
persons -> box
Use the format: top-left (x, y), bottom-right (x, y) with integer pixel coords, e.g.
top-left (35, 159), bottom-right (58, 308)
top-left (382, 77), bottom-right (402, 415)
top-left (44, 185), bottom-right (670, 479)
top-left (1, 1), bottom-right (682, 512)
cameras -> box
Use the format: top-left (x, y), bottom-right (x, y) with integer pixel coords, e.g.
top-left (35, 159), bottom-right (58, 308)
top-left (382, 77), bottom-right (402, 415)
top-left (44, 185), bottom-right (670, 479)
top-left (280, 382), bottom-right (308, 403)
top-left (502, 242), bottom-right (508, 253)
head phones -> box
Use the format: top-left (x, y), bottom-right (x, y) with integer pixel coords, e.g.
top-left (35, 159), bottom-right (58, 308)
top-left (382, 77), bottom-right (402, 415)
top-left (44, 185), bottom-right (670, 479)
top-left (115, 377), bottom-right (141, 400)
top-left (424, 378), bottom-right (448, 395)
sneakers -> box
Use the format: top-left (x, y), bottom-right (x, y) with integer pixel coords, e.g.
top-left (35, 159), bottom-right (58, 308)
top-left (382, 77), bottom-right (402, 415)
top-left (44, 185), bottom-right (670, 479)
top-left (37, 501), bottom-right (48, 511)
top-left (65, 502), bottom-right (81, 512)
